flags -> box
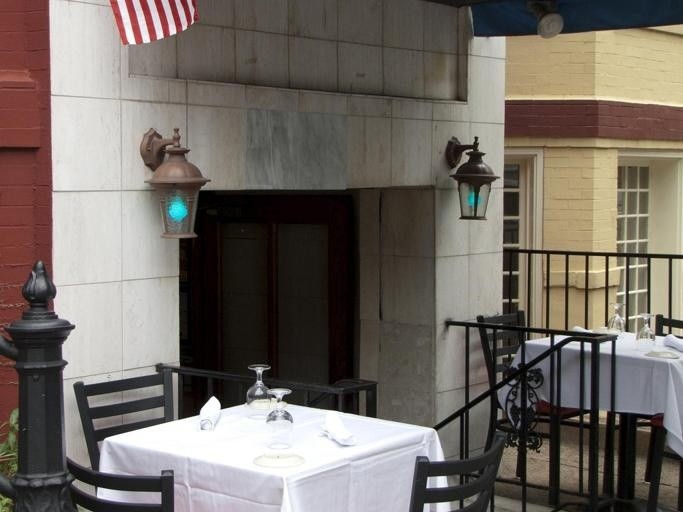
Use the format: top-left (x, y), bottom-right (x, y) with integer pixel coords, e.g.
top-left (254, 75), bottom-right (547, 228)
top-left (108, 0), bottom-right (200, 47)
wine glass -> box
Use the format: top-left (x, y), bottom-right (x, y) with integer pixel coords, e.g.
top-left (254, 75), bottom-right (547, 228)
top-left (607, 299), bottom-right (627, 336)
top-left (264, 387), bottom-right (295, 451)
top-left (244, 363), bottom-right (271, 421)
top-left (638, 312), bottom-right (656, 348)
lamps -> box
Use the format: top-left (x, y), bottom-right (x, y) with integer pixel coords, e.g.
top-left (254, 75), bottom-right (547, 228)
top-left (140, 125), bottom-right (212, 241)
top-left (445, 132), bottom-right (501, 223)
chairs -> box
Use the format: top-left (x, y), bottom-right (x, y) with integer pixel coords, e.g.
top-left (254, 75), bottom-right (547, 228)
top-left (66, 362), bottom-right (180, 509)
top-left (475, 308), bottom-right (589, 475)
top-left (411, 433), bottom-right (508, 512)
